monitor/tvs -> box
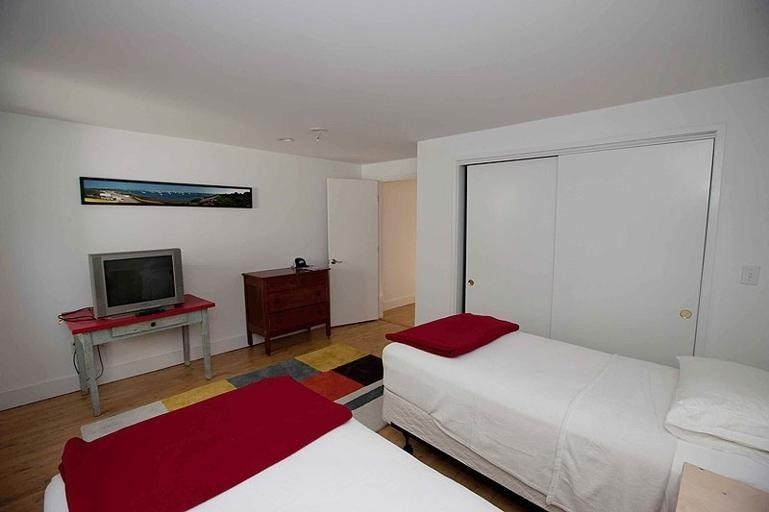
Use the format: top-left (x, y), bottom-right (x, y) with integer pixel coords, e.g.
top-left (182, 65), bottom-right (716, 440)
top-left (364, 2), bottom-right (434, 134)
top-left (89, 248), bottom-right (184, 321)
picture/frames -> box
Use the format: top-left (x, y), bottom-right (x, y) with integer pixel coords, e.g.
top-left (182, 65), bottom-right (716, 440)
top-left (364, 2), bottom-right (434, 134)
top-left (79, 176), bottom-right (253, 208)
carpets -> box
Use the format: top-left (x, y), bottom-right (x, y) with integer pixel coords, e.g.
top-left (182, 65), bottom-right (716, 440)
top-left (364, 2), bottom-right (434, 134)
top-left (80, 341), bottom-right (389, 443)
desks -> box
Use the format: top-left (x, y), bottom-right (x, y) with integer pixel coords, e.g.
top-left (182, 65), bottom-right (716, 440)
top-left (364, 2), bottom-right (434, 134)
top-left (61, 294), bottom-right (215, 417)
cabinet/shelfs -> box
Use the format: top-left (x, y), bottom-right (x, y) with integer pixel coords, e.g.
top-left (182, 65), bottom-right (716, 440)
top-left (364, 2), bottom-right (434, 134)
top-left (241, 265), bottom-right (331, 357)
top-left (450, 122), bottom-right (727, 367)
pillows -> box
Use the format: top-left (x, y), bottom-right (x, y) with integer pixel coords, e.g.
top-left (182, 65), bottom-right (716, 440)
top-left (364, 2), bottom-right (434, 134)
top-left (664, 353), bottom-right (768, 467)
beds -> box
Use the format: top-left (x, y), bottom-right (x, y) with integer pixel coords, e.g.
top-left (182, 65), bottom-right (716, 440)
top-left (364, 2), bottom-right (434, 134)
top-left (382, 313), bottom-right (769, 512)
top-left (43, 375), bottom-right (503, 512)
